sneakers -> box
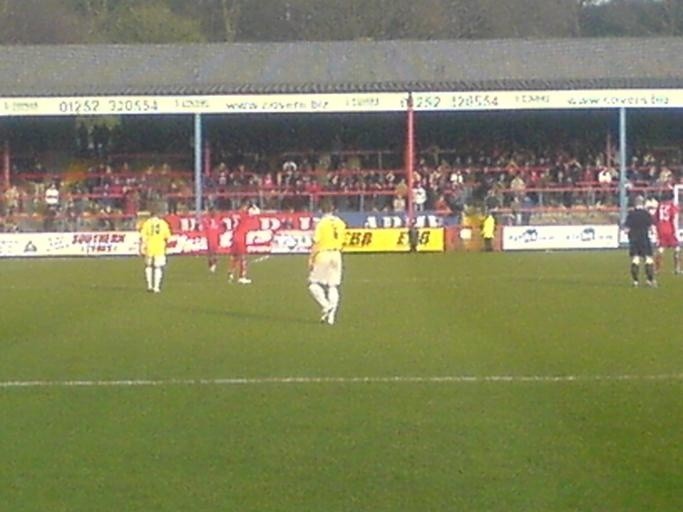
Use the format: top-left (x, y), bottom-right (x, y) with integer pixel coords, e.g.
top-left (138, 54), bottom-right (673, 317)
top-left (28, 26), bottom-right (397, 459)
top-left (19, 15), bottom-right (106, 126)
top-left (633, 280), bottom-right (656, 288)
top-left (228, 272), bottom-right (252, 284)
top-left (673, 267), bottom-right (683, 274)
top-left (209, 264), bottom-right (216, 272)
top-left (320, 305), bottom-right (336, 325)
top-left (148, 287), bottom-right (161, 293)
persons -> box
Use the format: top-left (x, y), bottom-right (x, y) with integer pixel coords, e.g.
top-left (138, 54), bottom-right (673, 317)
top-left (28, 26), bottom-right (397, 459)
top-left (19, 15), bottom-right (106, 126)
top-left (228, 203), bottom-right (259, 283)
top-left (0, 121), bottom-right (683, 233)
top-left (620, 199), bottom-right (656, 286)
top-left (307, 198), bottom-right (346, 325)
top-left (407, 221), bottom-right (419, 252)
top-left (205, 219), bottom-right (227, 274)
top-left (653, 192), bottom-right (683, 276)
top-left (481, 213), bottom-right (495, 251)
top-left (138, 205), bottom-right (172, 293)
